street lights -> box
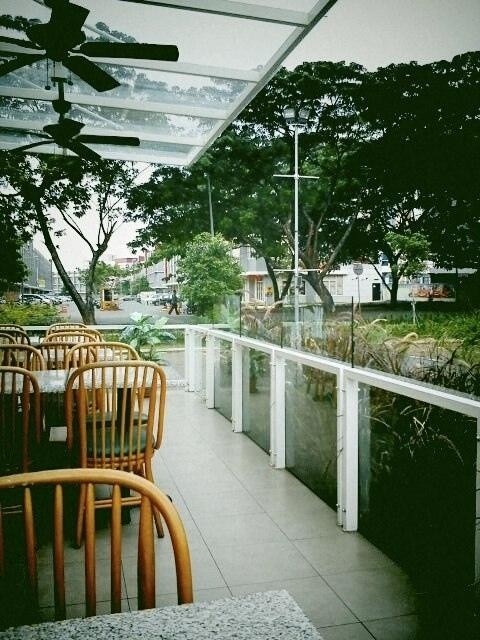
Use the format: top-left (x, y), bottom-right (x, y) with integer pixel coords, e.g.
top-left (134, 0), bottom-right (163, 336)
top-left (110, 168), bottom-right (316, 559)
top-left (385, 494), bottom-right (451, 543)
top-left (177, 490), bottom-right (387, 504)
top-left (272, 105), bottom-right (321, 387)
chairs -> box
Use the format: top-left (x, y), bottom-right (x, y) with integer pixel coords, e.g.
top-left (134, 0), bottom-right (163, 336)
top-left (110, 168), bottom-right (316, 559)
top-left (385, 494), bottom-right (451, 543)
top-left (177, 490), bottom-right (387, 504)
top-left (0, 322), bottom-right (166, 548)
top-left (0, 468), bottom-right (193, 628)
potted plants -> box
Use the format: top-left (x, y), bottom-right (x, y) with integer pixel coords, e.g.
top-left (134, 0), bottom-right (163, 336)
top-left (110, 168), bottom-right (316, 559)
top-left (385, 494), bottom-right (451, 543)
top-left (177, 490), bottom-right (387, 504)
top-left (250, 348), bottom-right (271, 393)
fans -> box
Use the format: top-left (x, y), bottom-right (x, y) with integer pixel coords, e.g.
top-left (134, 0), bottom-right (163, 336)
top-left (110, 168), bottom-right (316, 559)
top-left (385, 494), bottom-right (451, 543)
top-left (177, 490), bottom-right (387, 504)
top-left (0, 0), bottom-right (179, 93)
top-left (0, 77), bottom-right (140, 162)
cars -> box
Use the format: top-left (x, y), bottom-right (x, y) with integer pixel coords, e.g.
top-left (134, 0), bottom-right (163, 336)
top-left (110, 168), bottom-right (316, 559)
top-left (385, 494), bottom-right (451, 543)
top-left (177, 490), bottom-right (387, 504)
top-left (113, 295), bottom-right (138, 301)
top-left (19, 294), bottom-right (73, 306)
top-left (137, 291), bottom-right (184, 306)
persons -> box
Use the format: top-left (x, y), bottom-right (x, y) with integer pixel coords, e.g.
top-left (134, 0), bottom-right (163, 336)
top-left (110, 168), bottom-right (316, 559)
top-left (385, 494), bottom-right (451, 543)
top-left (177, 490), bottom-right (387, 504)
top-left (167, 289), bottom-right (181, 315)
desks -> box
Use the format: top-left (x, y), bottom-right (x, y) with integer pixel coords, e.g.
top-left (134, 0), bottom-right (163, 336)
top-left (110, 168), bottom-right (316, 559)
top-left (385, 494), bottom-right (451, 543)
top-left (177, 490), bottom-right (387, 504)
top-left (0, 589), bottom-right (324, 640)
